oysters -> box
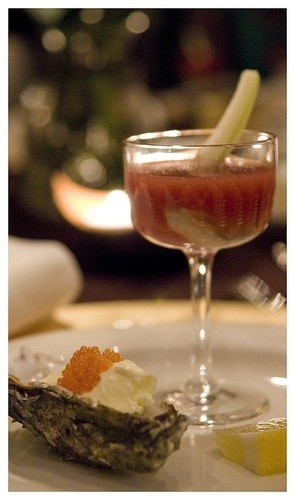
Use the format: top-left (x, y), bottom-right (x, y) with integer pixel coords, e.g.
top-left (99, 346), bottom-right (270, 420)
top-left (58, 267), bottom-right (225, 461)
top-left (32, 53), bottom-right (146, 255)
top-left (8, 345), bottom-right (190, 474)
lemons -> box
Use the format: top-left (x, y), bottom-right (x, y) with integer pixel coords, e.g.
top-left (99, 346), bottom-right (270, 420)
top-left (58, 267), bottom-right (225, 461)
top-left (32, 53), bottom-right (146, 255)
top-left (214, 416), bottom-right (287, 475)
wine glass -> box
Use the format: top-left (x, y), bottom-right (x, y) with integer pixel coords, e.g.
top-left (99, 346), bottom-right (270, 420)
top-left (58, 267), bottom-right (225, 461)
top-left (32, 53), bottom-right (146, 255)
top-left (123, 128), bottom-right (278, 426)
